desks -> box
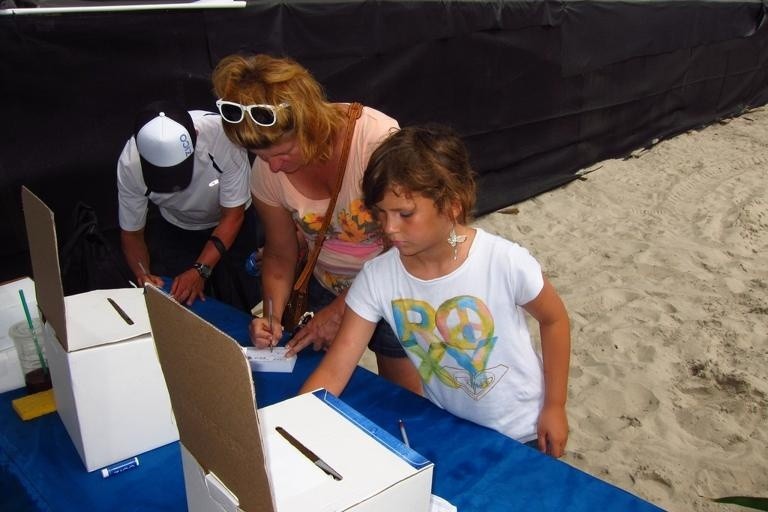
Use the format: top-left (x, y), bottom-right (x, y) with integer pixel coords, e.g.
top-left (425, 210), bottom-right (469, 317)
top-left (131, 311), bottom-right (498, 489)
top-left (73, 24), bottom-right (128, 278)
top-left (0, 275), bottom-right (671, 512)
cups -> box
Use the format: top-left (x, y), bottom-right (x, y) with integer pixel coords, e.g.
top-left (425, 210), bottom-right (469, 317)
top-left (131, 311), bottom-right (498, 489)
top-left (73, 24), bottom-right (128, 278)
top-left (8, 318), bottom-right (54, 395)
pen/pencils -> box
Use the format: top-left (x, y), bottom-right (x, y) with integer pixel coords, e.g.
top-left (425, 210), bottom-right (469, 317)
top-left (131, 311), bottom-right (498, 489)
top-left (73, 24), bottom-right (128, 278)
top-left (399, 419), bottom-right (410, 448)
top-left (267, 294), bottom-right (274, 353)
top-left (137, 261), bottom-right (153, 282)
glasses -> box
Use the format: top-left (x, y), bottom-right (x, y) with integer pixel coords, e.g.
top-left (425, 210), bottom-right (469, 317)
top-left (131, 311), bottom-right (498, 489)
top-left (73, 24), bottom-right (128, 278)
top-left (215, 97), bottom-right (291, 127)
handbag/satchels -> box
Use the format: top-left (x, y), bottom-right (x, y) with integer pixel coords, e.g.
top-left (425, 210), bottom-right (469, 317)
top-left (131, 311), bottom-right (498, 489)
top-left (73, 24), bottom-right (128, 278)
top-left (281, 288), bottom-right (310, 327)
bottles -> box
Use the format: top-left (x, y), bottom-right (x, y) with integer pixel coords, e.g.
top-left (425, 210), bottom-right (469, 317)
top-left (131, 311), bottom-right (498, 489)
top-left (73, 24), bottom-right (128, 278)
top-left (244, 231), bottom-right (303, 277)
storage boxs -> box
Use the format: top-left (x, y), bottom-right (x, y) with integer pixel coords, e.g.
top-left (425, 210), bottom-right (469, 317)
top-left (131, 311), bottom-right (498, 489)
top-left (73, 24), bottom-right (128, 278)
top-left (144, 283), bottom-right (434, 512)
top-left (17, 185), bottom-right (180, 473)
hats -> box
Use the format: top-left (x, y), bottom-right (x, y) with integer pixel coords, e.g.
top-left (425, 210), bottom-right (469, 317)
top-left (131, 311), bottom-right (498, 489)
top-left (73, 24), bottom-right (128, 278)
top-left (134, 99), bottom-right (196, 193)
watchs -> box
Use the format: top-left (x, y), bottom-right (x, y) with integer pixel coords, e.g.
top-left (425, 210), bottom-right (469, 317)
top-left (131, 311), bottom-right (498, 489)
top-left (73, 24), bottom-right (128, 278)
top-left (193, 259), bottom-right (214, 280)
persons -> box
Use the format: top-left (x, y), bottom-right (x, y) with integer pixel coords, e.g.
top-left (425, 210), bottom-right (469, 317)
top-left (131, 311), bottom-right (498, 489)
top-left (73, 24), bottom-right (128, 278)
top-left (296, 121), bottom-right (569, 457)
top-left (213, 54), bottom-right (424, 395)
top-left (118, 100), bottom-right (251, 305)
